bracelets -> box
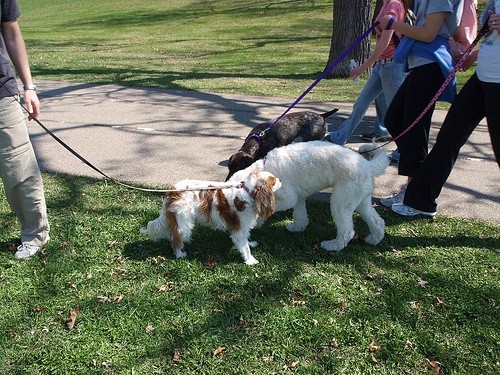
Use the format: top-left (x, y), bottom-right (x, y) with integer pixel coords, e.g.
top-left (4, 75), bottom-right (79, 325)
top-left (24, 85), bottom-right (37, 91)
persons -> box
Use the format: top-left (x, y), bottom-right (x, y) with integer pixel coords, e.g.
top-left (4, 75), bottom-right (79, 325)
top-left (324, 0), bottom-right (500, 216)
top-left (0, 0), bottom-right (51, 259)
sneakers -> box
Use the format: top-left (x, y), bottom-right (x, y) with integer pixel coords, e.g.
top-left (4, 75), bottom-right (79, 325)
top-left (391, 203), bottom-right (437, 216)
top-left (380, 187), bottom-right (407, 207)
top-left (389, 149), bottom-right (400, 161)
top-left (14, 236), bottom-right (50, 259)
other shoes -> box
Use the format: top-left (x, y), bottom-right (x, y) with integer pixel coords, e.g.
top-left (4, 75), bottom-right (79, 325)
top-left (362, 133), bottom-right (393, 142)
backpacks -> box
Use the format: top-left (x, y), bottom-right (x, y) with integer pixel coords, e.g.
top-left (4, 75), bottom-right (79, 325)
top-left (443, 0), bottom-right (478, 72)
top-left (393, 8), bottom-right (417, 48)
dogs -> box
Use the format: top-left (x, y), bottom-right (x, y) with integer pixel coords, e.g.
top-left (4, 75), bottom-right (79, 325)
top-left (224, 107), bottom-right (339, 181)
top-left (138, 168), bottom-right (282, 266)
top-left (226, 136), bottom-right (392, 252)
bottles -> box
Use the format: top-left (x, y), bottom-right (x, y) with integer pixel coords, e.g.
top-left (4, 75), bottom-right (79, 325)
top-left (350, 59), bottom-right (361, 84)
